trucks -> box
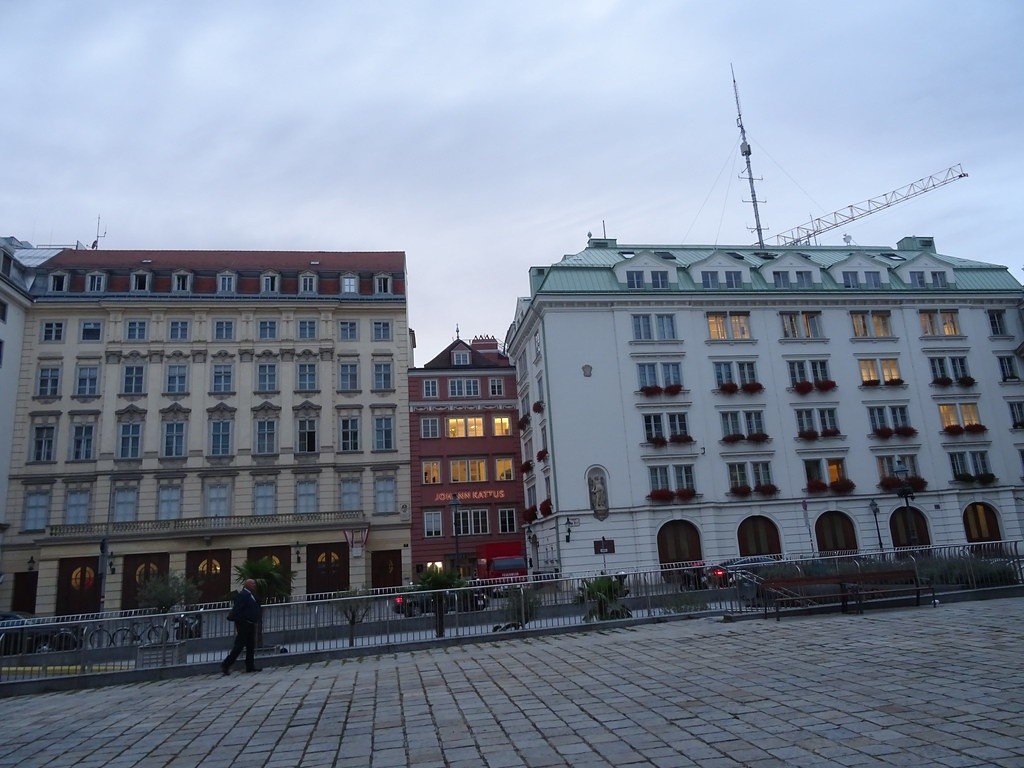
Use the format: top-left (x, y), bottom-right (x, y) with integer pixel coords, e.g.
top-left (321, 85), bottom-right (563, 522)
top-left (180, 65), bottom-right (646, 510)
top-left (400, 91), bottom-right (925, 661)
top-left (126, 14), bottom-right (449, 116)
top-left (478, 554), bottom-right (535, 597)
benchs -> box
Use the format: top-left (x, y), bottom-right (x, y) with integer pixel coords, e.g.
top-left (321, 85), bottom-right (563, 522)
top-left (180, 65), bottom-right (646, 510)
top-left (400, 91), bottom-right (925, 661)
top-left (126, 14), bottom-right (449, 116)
top-left (753, 569), bottom-right (936, 622)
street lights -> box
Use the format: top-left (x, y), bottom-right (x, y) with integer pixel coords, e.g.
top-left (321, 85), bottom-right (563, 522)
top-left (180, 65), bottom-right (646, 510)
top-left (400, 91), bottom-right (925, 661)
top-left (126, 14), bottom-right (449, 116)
top-left (870, 498), bottom-right (887, 563)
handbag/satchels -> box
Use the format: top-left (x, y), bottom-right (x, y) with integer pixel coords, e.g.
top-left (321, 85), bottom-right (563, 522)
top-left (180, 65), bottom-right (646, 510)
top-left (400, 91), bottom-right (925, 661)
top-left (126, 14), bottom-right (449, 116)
top-left (227, 599), bottom-right (261, 622)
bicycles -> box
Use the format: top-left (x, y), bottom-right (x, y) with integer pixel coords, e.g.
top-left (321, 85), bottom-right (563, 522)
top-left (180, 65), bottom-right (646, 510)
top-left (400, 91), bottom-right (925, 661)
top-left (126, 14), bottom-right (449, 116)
top-left (111, 616), bottom-right (170, 647)
top-left (49, 623), bottom-right (112, 653)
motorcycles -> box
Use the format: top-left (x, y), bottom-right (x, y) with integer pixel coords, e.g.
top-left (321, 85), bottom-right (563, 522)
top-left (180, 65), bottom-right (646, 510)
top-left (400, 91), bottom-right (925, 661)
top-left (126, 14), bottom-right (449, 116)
top-left (172, 607), bottom-right (204, 640)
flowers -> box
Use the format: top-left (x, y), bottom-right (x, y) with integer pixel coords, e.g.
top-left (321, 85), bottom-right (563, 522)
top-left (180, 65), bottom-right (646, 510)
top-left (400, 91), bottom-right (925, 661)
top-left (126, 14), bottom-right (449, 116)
top-left (521, 460), bottom-right (534, 474)
top-left (518, 412), bottom-right (531, 431)
top-left (647, 436), bottom-right (667, 448)
top-left (677, 489), bottom-right (696, 501)
top-left (669, 433), bottom-right (694, 444)
top-left (539, 498), bottom-right (553, 516)
top-left (533, 400), bottom-right (544, 414)
top-left (649, 488), bottom-right (675, 501)
top-left (536, 449), bottom-right (548, 462)
top-left (665, 383), bottom-right (683, 395)
top-left (720, 375), bottom-right (1024, 496)
top-left (639, 384), bottom-right (662, 396)
top-left (524, 504), bottom-right (537, 522)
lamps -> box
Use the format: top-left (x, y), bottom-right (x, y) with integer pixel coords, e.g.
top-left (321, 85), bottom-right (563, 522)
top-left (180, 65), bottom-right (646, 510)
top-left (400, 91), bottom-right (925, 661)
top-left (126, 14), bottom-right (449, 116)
top-left (565, 518), bottom-right (573, 542)
top-left (526, 527), bottom-right (532, 541)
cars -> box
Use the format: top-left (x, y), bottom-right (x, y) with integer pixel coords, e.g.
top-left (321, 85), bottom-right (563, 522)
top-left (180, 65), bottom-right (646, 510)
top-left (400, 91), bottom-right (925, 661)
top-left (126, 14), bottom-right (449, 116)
top-left (392, 581), bottom-right (491, 620)
top-left (0, 609), bottom-right (89, 656)
top-left (705, 556), bottom-right (802, 589)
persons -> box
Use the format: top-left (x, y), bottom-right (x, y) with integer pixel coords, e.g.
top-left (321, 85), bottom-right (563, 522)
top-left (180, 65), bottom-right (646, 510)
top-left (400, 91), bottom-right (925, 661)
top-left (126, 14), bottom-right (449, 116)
top-left (221, 578), bottom-right (264, 676)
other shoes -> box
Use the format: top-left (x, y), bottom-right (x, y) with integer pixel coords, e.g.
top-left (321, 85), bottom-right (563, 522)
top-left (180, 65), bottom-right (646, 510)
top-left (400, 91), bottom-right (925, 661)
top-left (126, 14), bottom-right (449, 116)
top-left (245, 667), bottom-right (262, 672)
top-left (222, 662), bottom-right (229, 675)
